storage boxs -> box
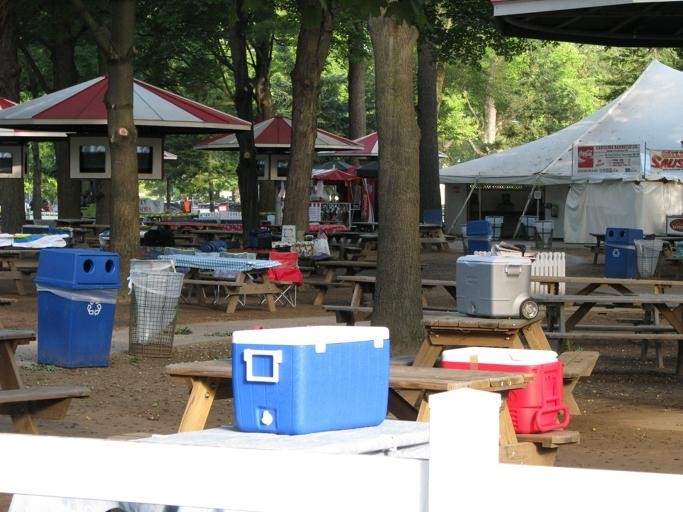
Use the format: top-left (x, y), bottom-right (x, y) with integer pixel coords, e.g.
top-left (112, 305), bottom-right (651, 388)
top-left (227, 325), bottom-right (389, 435)
top-left (439, 346), bottom-right (569, 434)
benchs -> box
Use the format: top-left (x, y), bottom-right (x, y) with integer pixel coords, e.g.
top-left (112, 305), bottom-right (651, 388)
top-left (539, 324), bottom-right (682, 377)
top-left (0, 386), bottom-right (91, 435)
top-left (558, 346), bottom-right (598, 416)
top-left (1, 222), bottom-right (457, 313)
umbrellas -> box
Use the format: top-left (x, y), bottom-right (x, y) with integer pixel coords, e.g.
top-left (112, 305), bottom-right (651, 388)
top-left (311, 157), bottom-right (379, 202)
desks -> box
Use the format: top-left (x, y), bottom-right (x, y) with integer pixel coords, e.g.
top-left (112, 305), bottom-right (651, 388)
top-left (410, 312), bottom-right (553, 368)
top-left (0, 327), bottom-right (36, 387)
top-left (588, 233), bottom-right (682, 265)
top-left (529, 276), bottom-right (682, 377)
top-left (162, 358), bottom-right (534, 447)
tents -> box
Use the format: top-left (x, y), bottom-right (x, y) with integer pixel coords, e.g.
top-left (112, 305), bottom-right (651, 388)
top-left (438, 56), bottom-right (683, 245)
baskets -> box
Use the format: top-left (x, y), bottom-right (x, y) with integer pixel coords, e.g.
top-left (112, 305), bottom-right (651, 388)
top-left (633, 239), bottom-right (664, 281)
top-left (535, 219), bottom-right (554, 250)
top-left (127, 270), bottom-right (185, 358)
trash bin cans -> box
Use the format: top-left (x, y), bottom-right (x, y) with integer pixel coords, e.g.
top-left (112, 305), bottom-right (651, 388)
top-left (466, 221), bottom-right (492, 255)
top-left (35, 248), bottom-right (123, 368)
top-left (604, 228), bottom-right (643, 278)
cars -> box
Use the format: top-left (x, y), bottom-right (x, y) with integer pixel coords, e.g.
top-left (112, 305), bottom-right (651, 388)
top-left (140, 196), bottom-right (185, 214)
top-left (218, 202), bottom-right (241, 212)
